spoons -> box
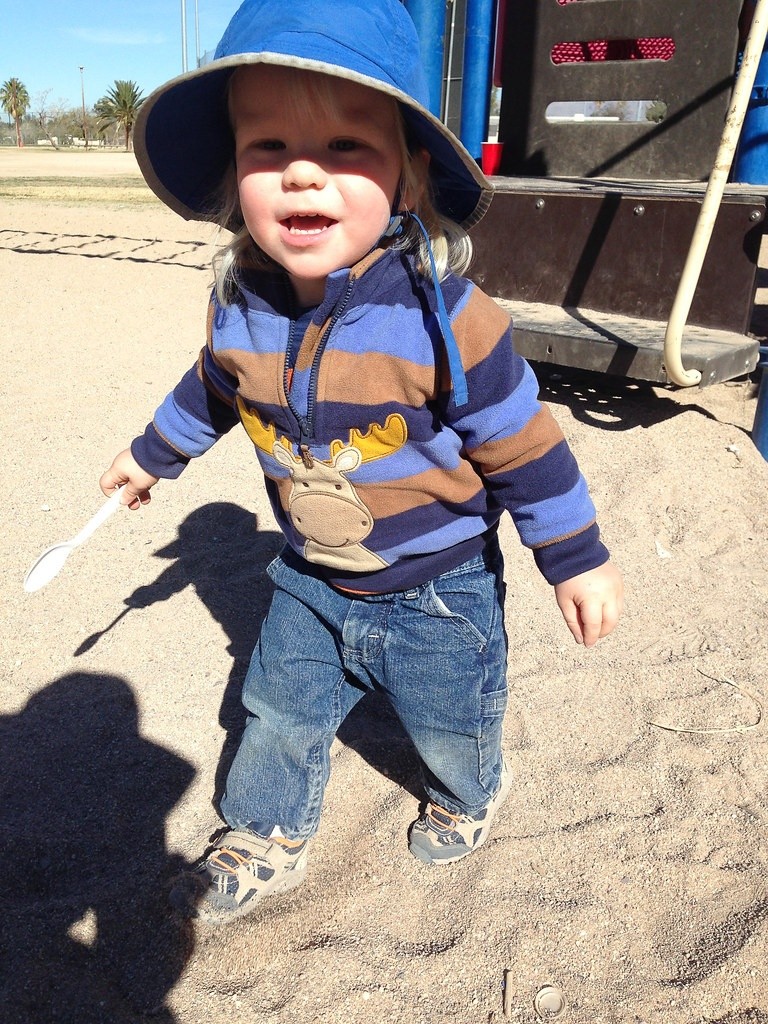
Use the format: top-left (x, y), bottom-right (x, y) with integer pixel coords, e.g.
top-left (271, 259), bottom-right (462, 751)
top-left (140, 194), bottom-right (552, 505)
top-left (24, 485), bottom-right (126, 591)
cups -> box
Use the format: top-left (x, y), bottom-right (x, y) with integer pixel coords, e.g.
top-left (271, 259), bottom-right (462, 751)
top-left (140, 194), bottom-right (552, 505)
top-left (481, 142), bottom-right (504, 176)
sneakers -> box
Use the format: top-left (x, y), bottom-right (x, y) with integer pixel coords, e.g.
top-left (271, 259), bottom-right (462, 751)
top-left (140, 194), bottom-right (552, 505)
top-left (169, 826), bottom-right (309, 923)
top-left (410, 758), bottom-right (514, 866)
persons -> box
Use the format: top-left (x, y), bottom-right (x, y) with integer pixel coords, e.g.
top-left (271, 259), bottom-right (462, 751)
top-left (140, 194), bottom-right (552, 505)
top-left (98, 0), bottom-right (622, 918)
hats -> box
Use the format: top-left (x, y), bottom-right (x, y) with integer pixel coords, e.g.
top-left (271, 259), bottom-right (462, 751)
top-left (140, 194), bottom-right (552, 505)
top-left (133, 0), bottom-right (495, 236)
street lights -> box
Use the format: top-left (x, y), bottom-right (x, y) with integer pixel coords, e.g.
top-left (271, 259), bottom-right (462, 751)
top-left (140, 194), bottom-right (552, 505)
top-left (77, 66), bottom-right (89, 148)
